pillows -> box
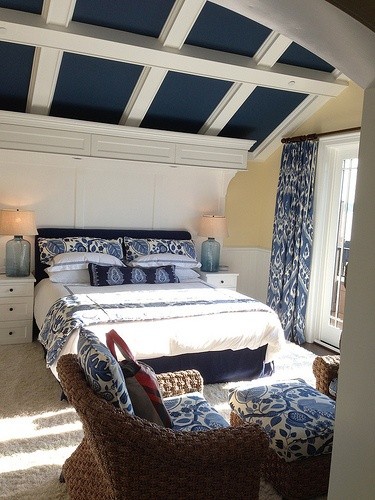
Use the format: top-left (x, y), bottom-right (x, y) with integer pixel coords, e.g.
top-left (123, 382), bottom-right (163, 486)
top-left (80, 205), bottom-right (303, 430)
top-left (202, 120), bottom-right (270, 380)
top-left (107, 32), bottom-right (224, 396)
top-left (45, 251), bottom-right (126, 273)
top-left (44, 269), bottom-right (91, 283)
top-left (130, 254), bottom-right (203, 270)
top-left (172, 267), bottom-right (201, 281)
top-left (73, 325), bottom-right (135, 417)
top-left (227, 378), bottom-right (336, 462)
top-left (88, 262), bottom-right (181, 288)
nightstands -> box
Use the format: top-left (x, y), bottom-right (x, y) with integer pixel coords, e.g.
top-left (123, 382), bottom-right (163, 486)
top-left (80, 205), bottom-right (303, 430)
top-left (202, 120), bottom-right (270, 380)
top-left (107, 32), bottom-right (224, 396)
top-left (194, 267), bottom-right (241, 292)
top-left (0, 272), bottom-right (39, 344)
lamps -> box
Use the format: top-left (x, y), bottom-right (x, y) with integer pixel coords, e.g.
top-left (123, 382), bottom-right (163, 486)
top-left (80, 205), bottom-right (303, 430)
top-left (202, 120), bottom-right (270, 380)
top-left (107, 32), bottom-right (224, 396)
top-left (0, 208), bottom-right (40, 279)
top-left (197, 215), bottom-right (230, 273)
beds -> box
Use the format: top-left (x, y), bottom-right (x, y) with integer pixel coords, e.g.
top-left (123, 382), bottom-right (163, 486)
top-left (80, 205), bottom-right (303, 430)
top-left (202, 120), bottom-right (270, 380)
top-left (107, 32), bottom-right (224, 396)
top-left (27, 228), bottom-right (287, 402)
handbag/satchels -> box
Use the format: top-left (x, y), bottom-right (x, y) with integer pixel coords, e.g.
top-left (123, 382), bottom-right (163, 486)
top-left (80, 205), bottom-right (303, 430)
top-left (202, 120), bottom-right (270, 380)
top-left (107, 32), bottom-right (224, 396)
top-left (105, 329), bottom-right (174, 429)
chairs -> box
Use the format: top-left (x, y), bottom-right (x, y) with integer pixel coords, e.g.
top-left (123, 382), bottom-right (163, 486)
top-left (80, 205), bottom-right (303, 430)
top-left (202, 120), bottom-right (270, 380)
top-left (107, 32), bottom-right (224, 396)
top-left (312, 355), bottom-right (342, 405)
top-left (56, 353), bottom-right (271, 500)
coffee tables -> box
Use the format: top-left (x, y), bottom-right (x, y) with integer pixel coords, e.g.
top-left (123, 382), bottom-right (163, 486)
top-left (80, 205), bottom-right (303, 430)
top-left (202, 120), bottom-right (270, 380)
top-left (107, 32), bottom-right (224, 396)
top-left (227, 411), bottom-right (337, 499)
